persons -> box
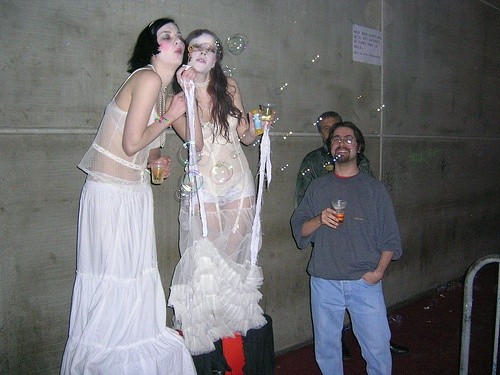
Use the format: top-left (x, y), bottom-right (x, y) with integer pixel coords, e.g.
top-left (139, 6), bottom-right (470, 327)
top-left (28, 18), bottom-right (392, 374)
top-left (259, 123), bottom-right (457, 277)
top-left (165, 27), bottom-right (275, 356)
top-left (289, 120), bottom-right (403, 375)
top-left (294, 110), bottom-right (410, 361)
top-left (61, 16), bottom-right (200, 373)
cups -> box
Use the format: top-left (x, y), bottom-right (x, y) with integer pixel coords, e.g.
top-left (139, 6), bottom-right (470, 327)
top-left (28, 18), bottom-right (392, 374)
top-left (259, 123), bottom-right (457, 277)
top-left (261, 103), bottom-right (275, 121)
top-left (330, 199), bottom-right (346, 225)
top-left (148, 163), bottom-right (168, 184)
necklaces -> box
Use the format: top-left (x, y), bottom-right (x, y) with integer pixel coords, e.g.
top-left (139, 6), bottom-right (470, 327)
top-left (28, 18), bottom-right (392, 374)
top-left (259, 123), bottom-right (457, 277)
top-left (195, 79), bottom-right (211, 87)
top-left (148, 64), bottom-right (166, 149)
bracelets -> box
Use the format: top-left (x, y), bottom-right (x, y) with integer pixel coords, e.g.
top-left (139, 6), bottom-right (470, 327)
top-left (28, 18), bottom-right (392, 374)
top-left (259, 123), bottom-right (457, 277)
top-left (153, 116), bottom-right (172, 129)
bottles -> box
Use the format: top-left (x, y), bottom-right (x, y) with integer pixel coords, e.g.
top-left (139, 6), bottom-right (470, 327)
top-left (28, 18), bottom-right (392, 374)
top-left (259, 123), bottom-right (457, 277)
top-left (251, 109), bottom-right (264, 135)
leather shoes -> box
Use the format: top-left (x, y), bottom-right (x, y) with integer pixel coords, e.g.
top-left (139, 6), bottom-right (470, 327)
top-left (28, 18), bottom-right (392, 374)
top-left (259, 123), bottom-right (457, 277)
top-left (341, 340), bottom-right (352, 360)
top-left (389, 341), bottom-right (410, 356)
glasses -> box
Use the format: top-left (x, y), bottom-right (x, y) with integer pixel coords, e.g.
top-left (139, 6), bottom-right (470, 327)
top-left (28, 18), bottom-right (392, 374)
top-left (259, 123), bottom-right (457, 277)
top-left (330, 135), bottom-right (358, 144)
